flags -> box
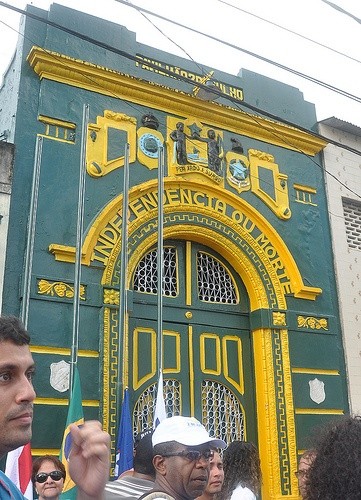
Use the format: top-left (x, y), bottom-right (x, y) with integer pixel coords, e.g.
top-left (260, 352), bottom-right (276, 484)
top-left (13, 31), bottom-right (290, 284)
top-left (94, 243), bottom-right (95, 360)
top-left (153, 372), bottom-right (166, 431)
top-left (114, 388), bottom-right (134, 479)
top-left (4, 442), bottom-right (34, 500)
top-left (58, 365), bottom-right (85, 500)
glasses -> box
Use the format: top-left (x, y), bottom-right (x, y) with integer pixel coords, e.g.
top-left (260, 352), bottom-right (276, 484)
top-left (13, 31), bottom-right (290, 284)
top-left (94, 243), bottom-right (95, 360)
top-left (32, 471), bottom-right (65, 483)
top-left (160, 451), bottom-right (214, 462)
top-left (294, 470), bottom-right (309, 480)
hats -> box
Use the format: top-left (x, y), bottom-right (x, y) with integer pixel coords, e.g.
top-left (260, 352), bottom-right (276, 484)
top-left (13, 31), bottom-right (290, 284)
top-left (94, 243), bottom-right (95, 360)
top-left (133, 429), bottom-right (153, 450)
top-left (151, 416), bottom-right (226, 449)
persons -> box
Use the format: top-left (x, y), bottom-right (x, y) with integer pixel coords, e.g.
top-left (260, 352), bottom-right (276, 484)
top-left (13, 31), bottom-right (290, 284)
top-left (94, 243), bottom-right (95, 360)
top-left (0, 312), bottom-right (111, 500)
top-left (137, 415), bottom-right (226, 500)
top-left (170, 122), bottom-right (190, 165)
top-left (31, 454), bottom-right (66, 500)
top-left (194, 450), bottom-right (224, 500)
top-left (194, 129), bottom-right (222, 175)
top-left (100, 433), bottom-right (156, 500)
top-left (213, 441), bottom-right (263, 500)
top-left (294, 410), bottom-right (361, 500)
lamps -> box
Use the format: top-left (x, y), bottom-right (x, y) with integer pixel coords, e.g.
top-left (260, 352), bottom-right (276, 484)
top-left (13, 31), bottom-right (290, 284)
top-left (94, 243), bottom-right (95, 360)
top-left (198, 78), bottom-right (221, 101)
